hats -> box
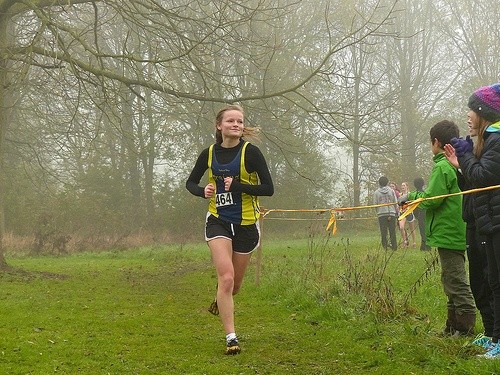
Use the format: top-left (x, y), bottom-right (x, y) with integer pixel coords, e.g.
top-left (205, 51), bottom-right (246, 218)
top-left (467, 83), bottom-right (500, 121)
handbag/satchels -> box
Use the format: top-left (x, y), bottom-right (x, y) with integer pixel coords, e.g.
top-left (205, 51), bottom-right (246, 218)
top-left (405, 213), bottom-right (413, 221)
top-left (413, 207), bottom-right (421, 219)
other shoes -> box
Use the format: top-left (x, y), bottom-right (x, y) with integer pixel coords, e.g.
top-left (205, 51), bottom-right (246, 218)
top-left (413, 243), bottom-right (416, 249)
top-left (420, 245), bottom-right (432, 251)
top-left (402, 241), bottom-right (409, 249)
top-left (440, 327), bottom-right (455, 337)
top-left (450, 330), bottom-right (469, 339)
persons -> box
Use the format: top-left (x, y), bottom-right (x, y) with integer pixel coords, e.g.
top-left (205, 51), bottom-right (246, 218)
top-left (443, 117), bottom-right (494, 350)
top-left (185, 105), bottom-right (275, 356)
top-left (398, 182), bottom-right (417, 248)
top-left (388, 183), bottom-right (402, 200)
top-left (449, 84), bottom-right (500, 360)
top-left (398, 120), bottom-right (476, 344)
top-left (408, 177), bottom-right (433, 251)
top-left (374, 177), bottom-right (399, 251)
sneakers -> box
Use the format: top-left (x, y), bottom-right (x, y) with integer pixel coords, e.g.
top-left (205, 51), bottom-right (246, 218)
top-left (472, 336), bottom-right (500, 359)
top-left (225, 338), bottom-right (241, 355)
top-left (208, 298), bottom-right (219, 316)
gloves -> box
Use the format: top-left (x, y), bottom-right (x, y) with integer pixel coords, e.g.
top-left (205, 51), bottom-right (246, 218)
top-left (450, 135), bottom-right (473, 157)
